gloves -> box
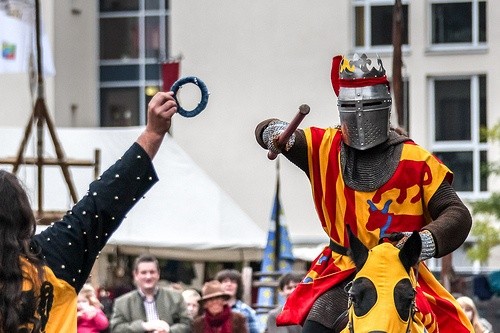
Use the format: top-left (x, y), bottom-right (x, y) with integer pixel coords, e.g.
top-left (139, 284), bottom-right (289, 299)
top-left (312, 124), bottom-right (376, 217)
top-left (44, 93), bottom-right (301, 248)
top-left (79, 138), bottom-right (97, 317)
top-left (389, 229), bottom-right (435, 263)
top-left (263, 119), bottom-right (296, 154)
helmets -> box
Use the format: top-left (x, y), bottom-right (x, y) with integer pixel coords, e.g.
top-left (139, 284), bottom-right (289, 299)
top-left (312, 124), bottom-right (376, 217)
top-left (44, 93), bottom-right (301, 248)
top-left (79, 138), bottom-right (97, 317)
top-left (337, 51), bottom-right (392, 149)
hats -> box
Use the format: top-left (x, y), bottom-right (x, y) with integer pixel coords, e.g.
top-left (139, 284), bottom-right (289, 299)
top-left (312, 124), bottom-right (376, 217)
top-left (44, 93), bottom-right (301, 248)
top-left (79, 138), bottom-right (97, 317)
top-left (197, 279), bottom-right (233, 303)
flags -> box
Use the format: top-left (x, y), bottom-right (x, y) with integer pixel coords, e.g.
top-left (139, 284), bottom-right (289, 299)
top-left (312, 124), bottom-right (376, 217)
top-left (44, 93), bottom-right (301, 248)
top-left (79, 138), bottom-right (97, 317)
top-left (255, 170), bottom-right (296, 333)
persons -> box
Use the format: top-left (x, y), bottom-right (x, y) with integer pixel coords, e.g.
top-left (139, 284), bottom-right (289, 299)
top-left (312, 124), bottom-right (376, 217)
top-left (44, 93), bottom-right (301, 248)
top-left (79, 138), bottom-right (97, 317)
top-left (268, 274), bottom-right (304, 333)
top-left (0, 91), bottom-right (176, 333)
top-left (76, 253), bottom-right (256, 333)
top-left (254, 50), bottom-right (475, 333)
top-left (450, 275), bottom-right (500, 333)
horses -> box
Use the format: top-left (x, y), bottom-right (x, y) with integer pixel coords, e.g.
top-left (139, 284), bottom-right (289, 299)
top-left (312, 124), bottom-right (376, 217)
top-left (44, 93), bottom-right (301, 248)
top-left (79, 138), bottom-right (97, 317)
top-left (338, 223), bottom-right (430, 333)
